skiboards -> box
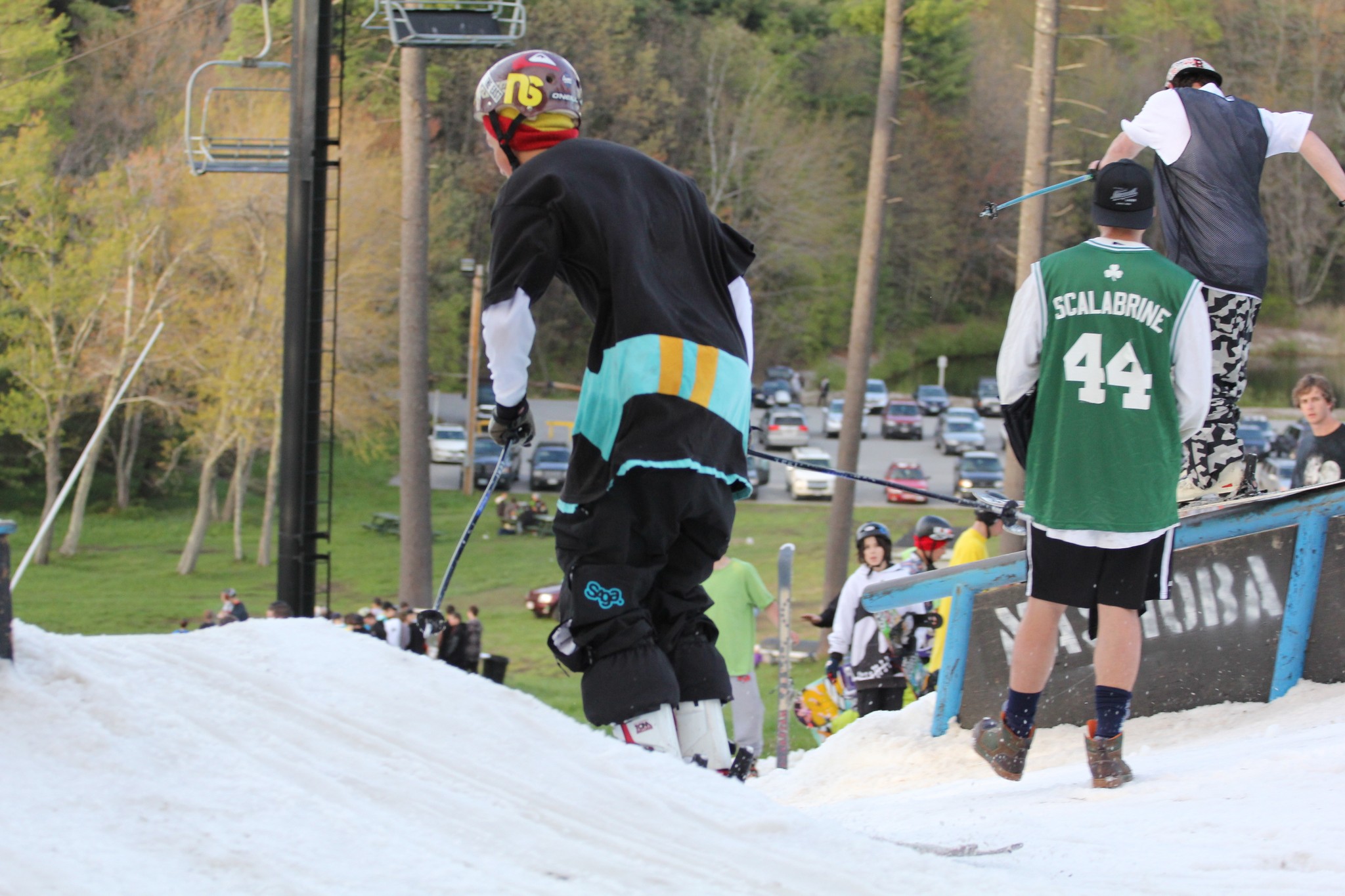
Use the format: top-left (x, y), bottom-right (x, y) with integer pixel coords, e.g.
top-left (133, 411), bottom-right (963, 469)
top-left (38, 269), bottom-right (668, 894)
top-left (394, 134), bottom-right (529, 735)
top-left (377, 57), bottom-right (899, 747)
top-left (972, 477), bottom-right (1345, 536)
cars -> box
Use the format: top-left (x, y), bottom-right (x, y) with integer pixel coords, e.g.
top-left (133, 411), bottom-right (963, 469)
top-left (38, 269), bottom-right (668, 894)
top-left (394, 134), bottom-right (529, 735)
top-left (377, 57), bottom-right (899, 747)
top-left (1235, 414), bottom-right (1276, 460)
top-left (973, 375), bottom-right (1006, 416)
top-left (756, 364), bottom-right (799, 407)
top-left (933, 405), bottom-right (986, 456)
top-left (525, 583), bottom-right (562, 621)
top-left (913, 382), bottom-right (953, 416)
top-left (526, 439), bottom-right (571, 492)
top-left (426, 423), bottom-right (471, 463)
top-left (884, 462), bottom-right (933, 504)
top-left (953, 451), bottom-right (1006, 501)
top-left (822, 399), bottom-right (867, 439)
top-left (860, 379), bottom-right (923, 440)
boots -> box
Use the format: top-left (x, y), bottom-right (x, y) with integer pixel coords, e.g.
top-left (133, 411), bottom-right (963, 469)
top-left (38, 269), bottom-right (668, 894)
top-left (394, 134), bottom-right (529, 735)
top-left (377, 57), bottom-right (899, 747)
top-left (974, 701), bottom-right (1035, 781)
top-left (1083, 717), bottom-right (1132, 789)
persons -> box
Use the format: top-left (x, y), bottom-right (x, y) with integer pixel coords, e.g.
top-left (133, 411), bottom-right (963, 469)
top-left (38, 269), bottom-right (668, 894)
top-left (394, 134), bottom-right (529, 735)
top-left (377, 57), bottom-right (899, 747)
top-left (266, 600), bottom-right (292, 619)
top-left (971, 160), bottom-right (1213, 786)
top-left (914, 492), bottom-right (1008, 699)
top-left (474, 50), bottom-right (755, 775)
top-left (699, 555), bottom-right (799, 757)
top-left (199, 611), bottom-right (215, 629)
top-left (1086, 56), bottom-right (1345, 502)
top-left (217, 589), bottom-right (248, 624)
top-left (791, 373), bottom-right (803, 403)
top-left (447, 614), bottom-right (470, 670)
top-left (1290, 373), bottom-right (1345, 489)
top-left (801, 516), bottom-right (955, 629)
top-left (437, 605), bottom-right (455, 659)
top-left (334, 597), bottom-right (426, 654)
top-left (825, 523), bottom-right (936, 717)
top-left (817, 376), bottom-right (830, 406)
top-left (464, 606), bottom-right (482, 673)
top-left (172, 618), bottom-right (189, 634)
top-left (495, 491), bottom-right (548, 535)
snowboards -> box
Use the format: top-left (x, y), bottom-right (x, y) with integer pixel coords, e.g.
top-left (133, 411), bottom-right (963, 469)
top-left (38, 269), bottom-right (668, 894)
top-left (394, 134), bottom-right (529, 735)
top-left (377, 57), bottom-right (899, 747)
top-left (874, 608), bottom-right (936, 702)
top-left (793, 627), bottom-right (935, 728)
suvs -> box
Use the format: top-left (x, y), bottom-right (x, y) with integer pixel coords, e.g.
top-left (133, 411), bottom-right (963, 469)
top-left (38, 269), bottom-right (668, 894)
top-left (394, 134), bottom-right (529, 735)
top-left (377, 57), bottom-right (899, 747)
top-left (469, 415), bottom-right (524, 490)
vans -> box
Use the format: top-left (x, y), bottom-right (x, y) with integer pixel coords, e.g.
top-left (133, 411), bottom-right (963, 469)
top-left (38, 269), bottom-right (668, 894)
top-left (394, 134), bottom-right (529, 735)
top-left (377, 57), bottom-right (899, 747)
top-left (757, 407), bottom-right (809, 450)
top-left (1256, 458), bottom-right (1299, 494)
top-left (784, 446), bottom-right (838, 500)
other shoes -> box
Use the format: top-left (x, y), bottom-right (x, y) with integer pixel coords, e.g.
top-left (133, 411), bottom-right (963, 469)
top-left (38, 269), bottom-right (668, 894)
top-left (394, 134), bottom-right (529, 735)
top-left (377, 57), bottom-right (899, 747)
top-left (1211, 460), bottom-right (1245, 495)
top-left (1176, 479), bottom-right (1205, 503)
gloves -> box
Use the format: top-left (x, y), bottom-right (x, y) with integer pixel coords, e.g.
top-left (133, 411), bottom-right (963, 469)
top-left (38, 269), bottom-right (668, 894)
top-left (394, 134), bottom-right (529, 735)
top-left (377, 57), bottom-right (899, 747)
top-left (487, 401), bottom-right (536, 449)
top-left (824, 652), bottom-right (842, 683)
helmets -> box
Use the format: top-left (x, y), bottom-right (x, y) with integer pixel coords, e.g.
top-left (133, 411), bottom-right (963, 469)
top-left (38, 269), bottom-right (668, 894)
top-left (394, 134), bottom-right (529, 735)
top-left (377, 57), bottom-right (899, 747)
top-left (915, 515), bottom-right (953, 543)
top-left (856, 522), bottom-right (891, 547)
top-left (1165, 57), bottom-right (1222, 93)
top-left (976, 493), bottom-right (1007, 518)
top-left (474, 49), bottom-right (583, 131)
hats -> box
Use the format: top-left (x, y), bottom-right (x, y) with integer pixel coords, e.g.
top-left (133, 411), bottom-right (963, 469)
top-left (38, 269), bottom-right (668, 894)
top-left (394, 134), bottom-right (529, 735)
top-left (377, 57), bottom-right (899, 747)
top-left (483, 104), bottom-right (580, 151)
top-left (914, 535), bottom-right (947, 551)
top-left (1091, 159), bottom-right (1155, 229)
